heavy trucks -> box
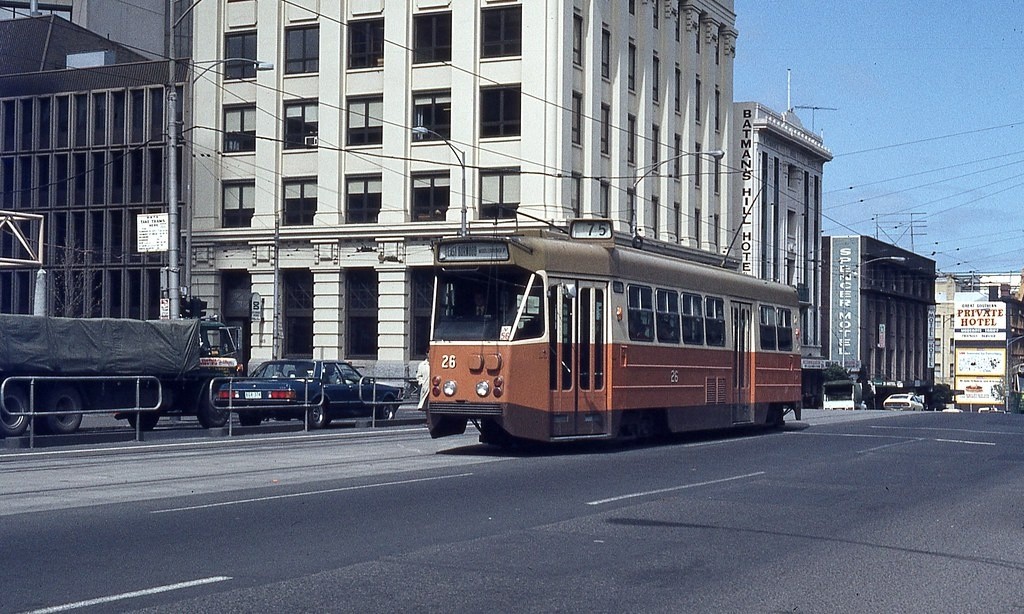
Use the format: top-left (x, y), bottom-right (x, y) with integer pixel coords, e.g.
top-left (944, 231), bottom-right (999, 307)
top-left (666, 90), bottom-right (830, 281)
top-left (0, 311), bottom-right (238, 436)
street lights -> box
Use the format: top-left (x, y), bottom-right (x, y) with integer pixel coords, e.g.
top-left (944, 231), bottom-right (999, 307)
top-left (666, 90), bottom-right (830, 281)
top-left (841, 256), bottom-right (905, 371)
top-left (184, 56), bottom-right (276, 302)
top-left (1005, 336), bottom-right (1024, 414)
top-left (411, 124), bottom-right (468, 238)
top-left (632, 149), bottom-right (727, 237)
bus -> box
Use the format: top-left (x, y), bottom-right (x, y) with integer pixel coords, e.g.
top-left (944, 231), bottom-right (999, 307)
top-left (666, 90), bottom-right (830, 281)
top-left (414, 218), bottom-right (804, 452)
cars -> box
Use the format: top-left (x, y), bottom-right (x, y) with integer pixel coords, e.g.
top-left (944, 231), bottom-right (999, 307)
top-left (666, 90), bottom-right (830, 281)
top-left (883, 393), bottom-right (924, 412)
top-left (938, 405), bottom-right (1007, 414)
top-left (216, 359), bottom-right (405, 429)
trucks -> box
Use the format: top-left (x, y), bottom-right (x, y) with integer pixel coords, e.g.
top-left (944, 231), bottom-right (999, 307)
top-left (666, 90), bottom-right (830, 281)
top-left (822, 379), bottom-right (863, 412)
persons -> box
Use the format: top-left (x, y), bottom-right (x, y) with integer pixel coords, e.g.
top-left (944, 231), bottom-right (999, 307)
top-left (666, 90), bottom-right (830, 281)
top-left (455, 291), bottom-right (496, 322)
top-left (629, 310), bottom-right (792, 352)
top-left (855, 399), bottom-right (868, 411)
top-left (293, 363), bottom-right (336, 386)
